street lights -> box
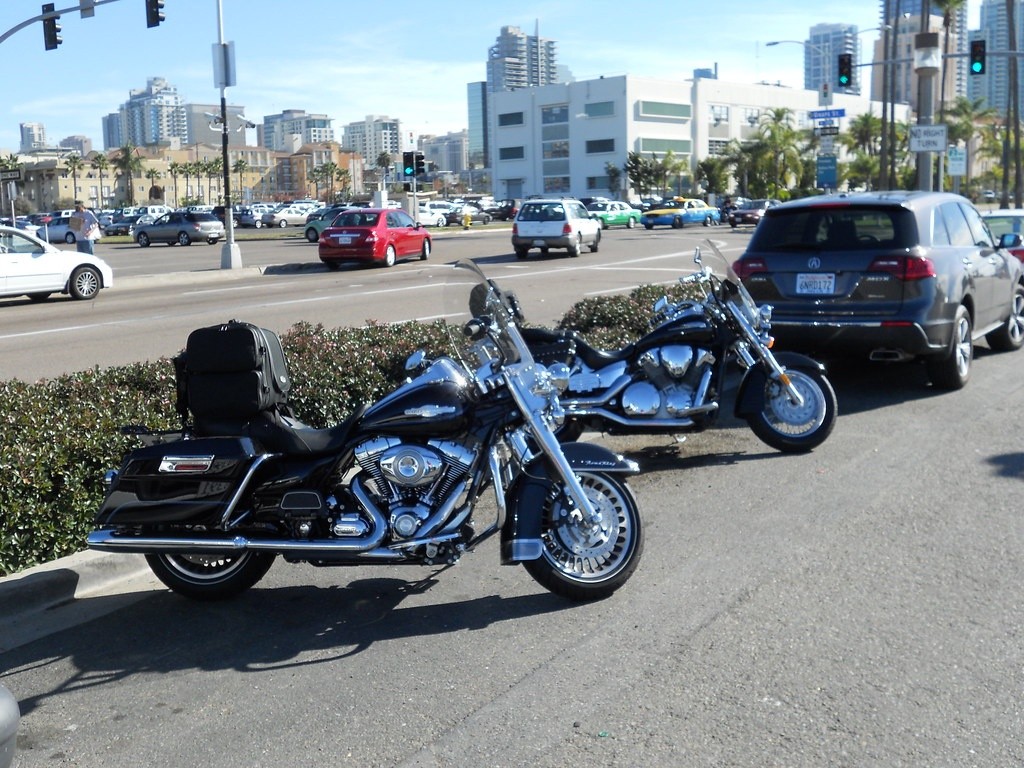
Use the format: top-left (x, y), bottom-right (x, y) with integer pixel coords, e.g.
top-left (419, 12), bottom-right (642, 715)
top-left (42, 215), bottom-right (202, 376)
top-left (766, 25), bottom-right (892, 195)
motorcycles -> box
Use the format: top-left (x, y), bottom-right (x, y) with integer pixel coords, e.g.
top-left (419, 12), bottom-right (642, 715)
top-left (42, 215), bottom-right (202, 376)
top-left (86, 260), bottom-right (646, 600)
top-left (518, 238), bottom-right (838, 453)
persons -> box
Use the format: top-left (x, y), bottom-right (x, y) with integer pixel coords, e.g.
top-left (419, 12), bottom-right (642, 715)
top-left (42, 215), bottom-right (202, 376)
top-left (69, 198), bottom-right (99, 255)
top-left (463, 213), bottom-right (471, 227)
top-left (720, 193), bottom-right (730, 207)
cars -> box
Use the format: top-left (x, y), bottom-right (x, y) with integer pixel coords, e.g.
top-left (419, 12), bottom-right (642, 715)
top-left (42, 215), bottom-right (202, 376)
top-left (304, 200), bottom-right (522, 242)
top-left (176, 201), bottom-right (325, 228)
top-left (104, 214), bottom-right (166, 236)
top-left (132, 211), bottom-right (225, 247)
top-left (36, 217), bottom-right (101, 244)
top-left (318, 208), bottom-right (432, 269)
top-left (0, 226), bottom-right (113, 300)
top-left (583, 197), bottom-right (782, 229)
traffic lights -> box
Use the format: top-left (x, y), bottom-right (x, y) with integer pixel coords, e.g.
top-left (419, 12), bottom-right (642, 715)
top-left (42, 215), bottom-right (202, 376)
top-left (838, 54), bottom-right (852, 86)
top-left (970, 40), bottom-right (986, 75)
top-left (403, 151), bottom-right (425, 176)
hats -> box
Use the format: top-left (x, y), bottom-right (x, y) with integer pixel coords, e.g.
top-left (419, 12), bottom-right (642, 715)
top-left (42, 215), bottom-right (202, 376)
top-left (74, 200), bottom-right (84, 207)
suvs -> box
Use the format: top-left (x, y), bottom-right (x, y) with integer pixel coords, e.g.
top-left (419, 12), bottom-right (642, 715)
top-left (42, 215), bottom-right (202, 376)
top-left (724, 190), bottom-right (1024, 389)
top-left (511, 195), bottom-right (601, 258)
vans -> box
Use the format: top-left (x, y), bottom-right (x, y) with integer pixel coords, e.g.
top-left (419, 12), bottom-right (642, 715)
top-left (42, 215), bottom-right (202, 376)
top-left (0, 205), bottom-right (175, 230)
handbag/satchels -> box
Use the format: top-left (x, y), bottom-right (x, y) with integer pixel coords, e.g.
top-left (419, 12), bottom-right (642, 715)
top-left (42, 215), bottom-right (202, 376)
top-left (186, 319), bottom-right (290, 419)
